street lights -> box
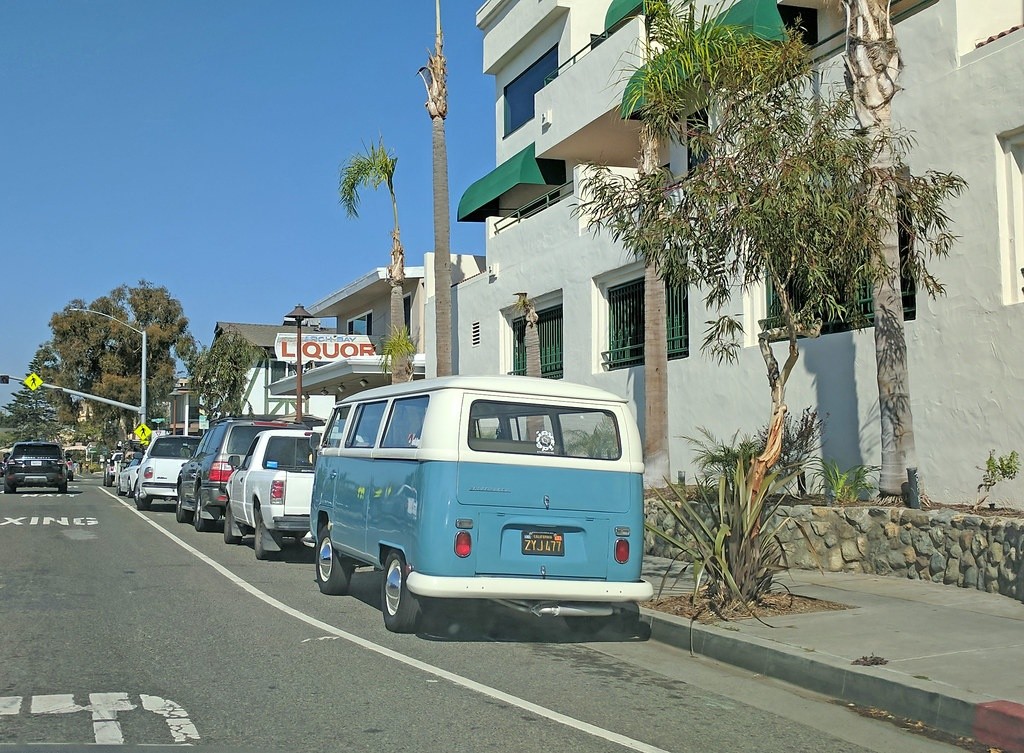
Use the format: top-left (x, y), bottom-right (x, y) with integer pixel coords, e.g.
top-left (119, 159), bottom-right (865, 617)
top-left (70, 308), bottom-right (146, 425)
top-left (284, 304), bottom-right (315, 423)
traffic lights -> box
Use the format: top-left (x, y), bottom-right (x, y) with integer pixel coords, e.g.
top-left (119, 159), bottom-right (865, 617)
top-left (0, 375), bottom-right (9, 384)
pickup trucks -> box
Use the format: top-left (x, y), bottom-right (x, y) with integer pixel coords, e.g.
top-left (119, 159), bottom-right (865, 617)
top-left (133, 435), bottom-right (202, 510)
top-left (102, 453), bottom-right (122, 487)
top-left (225, 429), bottom-right (365, 560)
top-left (3, 442), bottom-right (69, 494)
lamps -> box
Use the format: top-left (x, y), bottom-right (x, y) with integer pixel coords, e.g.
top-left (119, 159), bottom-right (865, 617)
top-left (304, 392), bottom-right (310, 400)
top-left (338, 383), bottom-right (345, 392)
top-left (320, 387), bottom-right (329, 396)
top-left (360, 379), bottom-right (368, 387)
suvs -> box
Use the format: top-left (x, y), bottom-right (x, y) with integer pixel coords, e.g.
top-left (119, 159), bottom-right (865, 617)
top-left (176, 418), bottom-right (311, 532)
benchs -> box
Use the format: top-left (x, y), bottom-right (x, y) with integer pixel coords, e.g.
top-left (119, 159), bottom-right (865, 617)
top-left (468, 437), bottom-right (560, 456)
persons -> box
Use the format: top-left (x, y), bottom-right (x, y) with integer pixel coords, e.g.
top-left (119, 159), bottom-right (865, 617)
top-left (66, 459), bottom-right (83, 481)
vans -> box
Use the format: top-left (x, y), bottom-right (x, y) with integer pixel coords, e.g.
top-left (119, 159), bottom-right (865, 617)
top-left (310, 376), bottom-right (654, 635)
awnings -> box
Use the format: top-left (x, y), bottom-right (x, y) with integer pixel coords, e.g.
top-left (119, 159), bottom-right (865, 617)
top-left (604, 0), bottom-right (666, 34)
top-left (456, 141), bottom-right (566, 222)
top-left (621, 0), bottom-right (817, 124)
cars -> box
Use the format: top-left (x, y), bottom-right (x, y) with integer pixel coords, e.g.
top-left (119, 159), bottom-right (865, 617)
top-left (65, 453), bottom-right (74, 481)
top-left (115, 452), bottom-right (145, 498)
